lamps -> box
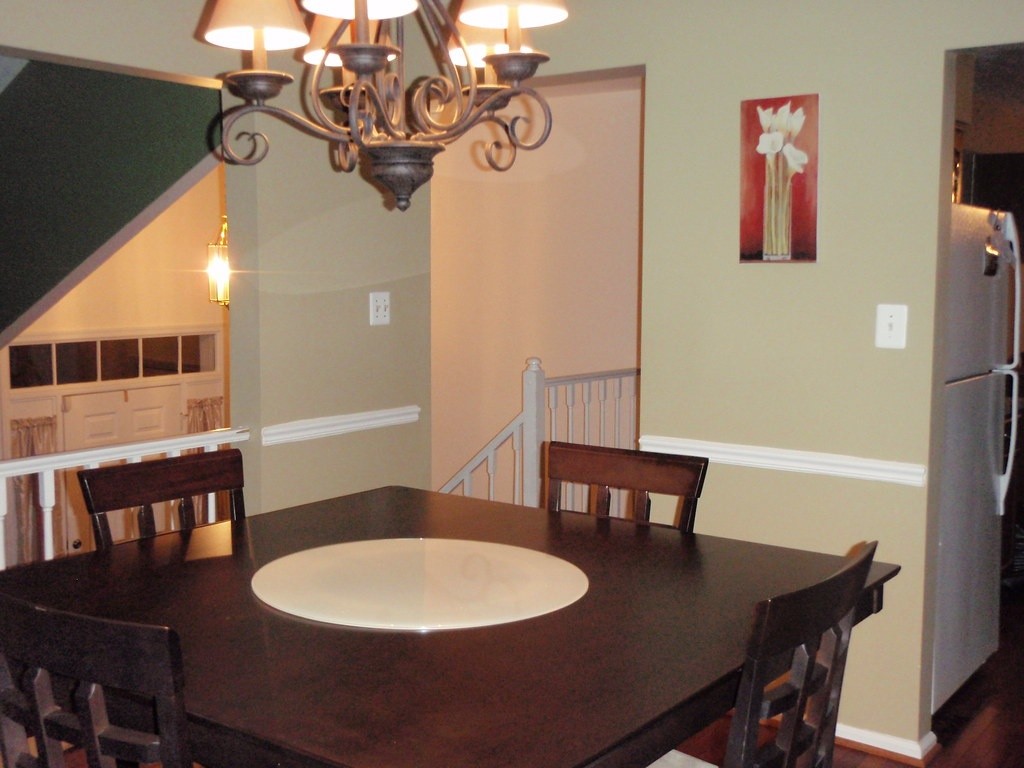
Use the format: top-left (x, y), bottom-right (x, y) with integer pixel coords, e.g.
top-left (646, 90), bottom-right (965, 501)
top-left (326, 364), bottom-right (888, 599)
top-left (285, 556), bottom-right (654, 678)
top-left (204, 0), bottom-right (568, 213)
top-left (207, 215), bottom-right (229, 310)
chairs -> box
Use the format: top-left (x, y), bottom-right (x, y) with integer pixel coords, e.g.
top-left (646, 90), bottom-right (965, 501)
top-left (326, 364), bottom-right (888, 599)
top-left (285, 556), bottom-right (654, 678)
top-left (723, 540), bottom-right (880, 767)
top-left (3, 595), bottom-right (191, 767)
top-left (540, 440), bottom-right (708, 531)
top-left (76, 448), bottom-right (246, 550)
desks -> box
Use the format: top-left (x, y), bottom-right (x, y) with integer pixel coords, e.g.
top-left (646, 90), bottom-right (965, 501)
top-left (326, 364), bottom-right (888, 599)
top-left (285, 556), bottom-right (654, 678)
top-left (1, 484), bottom-right (902, 768)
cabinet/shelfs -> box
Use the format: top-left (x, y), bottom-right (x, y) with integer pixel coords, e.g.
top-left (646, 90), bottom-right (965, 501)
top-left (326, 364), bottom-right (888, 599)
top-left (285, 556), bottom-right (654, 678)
top-left (1, 324), bottom-right (223, 571)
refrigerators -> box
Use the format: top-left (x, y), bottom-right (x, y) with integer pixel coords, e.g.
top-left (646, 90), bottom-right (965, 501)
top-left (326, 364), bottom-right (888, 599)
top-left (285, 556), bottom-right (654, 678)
top-left (931, 203), bottom-right (1019, 720)
